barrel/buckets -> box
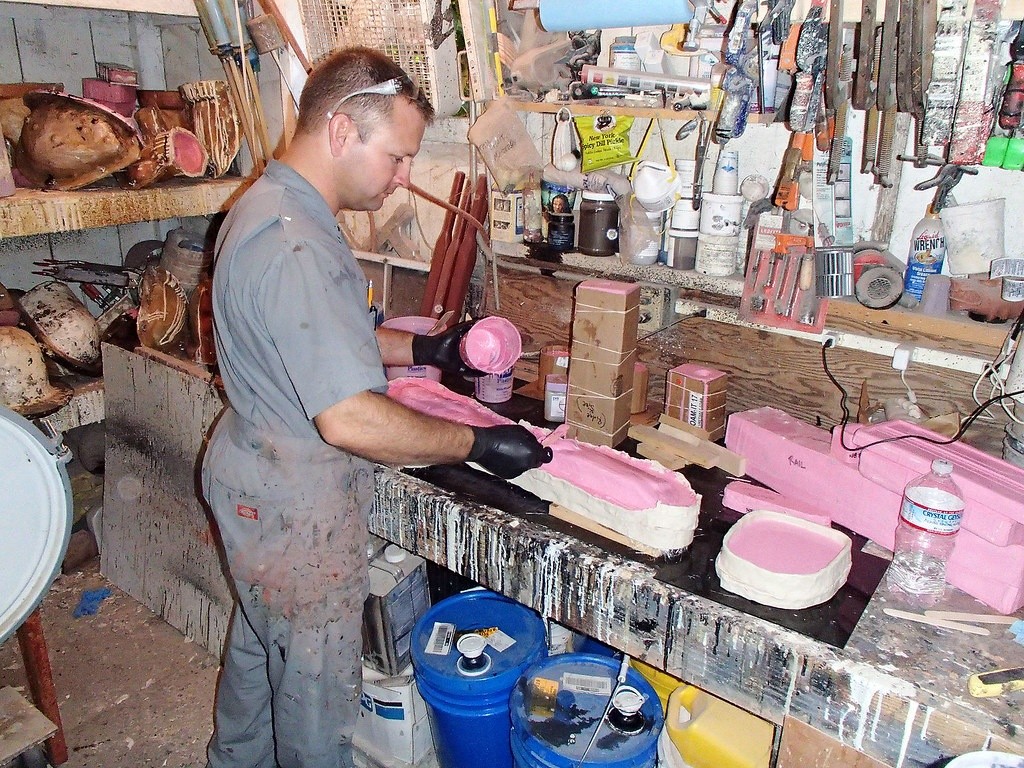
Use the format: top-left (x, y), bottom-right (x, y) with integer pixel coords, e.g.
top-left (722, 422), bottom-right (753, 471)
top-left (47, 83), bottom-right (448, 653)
top-left (411, 591), bottom-right (550, 768)
top-left (510, 654), bottom-right (666, 768)
top-left (658, 684), bottom-right (776, 768)
top-left (473, 367), bottom-right (514, 404)
top-left (541, 616), bottom-right (575, 659)
top-left (379, 316), bottom-right (447, 385)
top-left (628, 656), bottom-right (681, 716)
top-left (940, 196), bottom-right (1007, 274)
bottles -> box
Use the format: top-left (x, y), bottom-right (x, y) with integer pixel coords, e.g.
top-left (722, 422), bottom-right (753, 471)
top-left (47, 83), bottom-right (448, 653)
top-left (577, 186), bottom-right (616, 257)
top-left (665, 157), bottom-right (745, 278)
top-left (627, 211), bottom-right (660, 266)
top-left (889, 458), bottom-right (966, 601)
top-left (544, 213), bottom-right (575, 248)
top-left (609, 36), bottom-right (642, 75)
top-left (904, 203), bottom-right (947, 304)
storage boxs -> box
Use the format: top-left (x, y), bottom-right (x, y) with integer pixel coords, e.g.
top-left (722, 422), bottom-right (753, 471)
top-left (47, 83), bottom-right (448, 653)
top-left (664, 364), bottom-right (727, 442)
top-left (563, 279), bottom-right (641, 449)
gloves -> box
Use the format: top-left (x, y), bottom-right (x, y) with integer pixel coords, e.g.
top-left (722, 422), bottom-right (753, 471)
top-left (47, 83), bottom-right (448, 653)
top-left (412, 316), bottom-right (509, 378)
top-left (466, 424), bottom-right (545, 479)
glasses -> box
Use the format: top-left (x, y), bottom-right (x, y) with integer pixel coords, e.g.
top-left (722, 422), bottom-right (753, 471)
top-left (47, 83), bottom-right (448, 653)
top-left (325, 72), bottom-right (419, 121)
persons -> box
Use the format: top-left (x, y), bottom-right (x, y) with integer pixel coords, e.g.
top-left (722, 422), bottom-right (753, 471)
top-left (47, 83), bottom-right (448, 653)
top-left (552, 194), bottom-right (572, 215)
top-left (196, 46), bottom-right (553, 768)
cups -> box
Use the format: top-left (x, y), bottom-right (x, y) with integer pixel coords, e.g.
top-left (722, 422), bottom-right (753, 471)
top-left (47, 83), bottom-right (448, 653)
top-left (458, 316), bottom-right (523, 373)
top-left (712, 150), bottom-right (739, 195)
